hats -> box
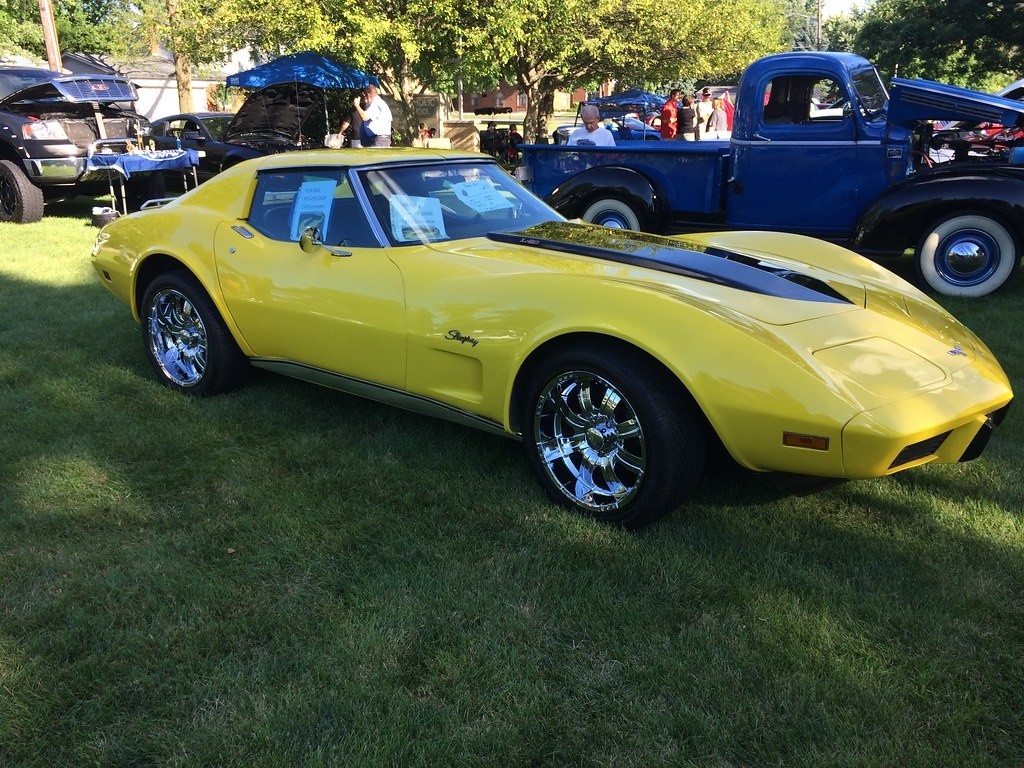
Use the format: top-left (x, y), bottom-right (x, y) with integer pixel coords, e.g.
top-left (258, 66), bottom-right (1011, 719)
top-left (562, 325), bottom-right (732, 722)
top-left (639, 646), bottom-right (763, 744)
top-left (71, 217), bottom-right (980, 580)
top-left (703, 88), bottom-right (712, 95)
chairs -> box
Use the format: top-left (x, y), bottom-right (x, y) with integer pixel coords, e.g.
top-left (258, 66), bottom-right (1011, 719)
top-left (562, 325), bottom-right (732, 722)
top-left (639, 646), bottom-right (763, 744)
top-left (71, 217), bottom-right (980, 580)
top-left (263, 205), bottom-right (292, 241)
top-left (769, 76), bottom-right (804, 125)
top-left (181, 120), bottom-right (197, 138)
top-left (333, 201), bottom-right (359, 244)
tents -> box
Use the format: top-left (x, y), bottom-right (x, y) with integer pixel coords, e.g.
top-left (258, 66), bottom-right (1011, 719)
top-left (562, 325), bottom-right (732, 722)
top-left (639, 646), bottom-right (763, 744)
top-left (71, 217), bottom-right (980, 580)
top-left (574, 88), bottom-right (669, 141)
top-left (220, 51), bottom-right (380, 152)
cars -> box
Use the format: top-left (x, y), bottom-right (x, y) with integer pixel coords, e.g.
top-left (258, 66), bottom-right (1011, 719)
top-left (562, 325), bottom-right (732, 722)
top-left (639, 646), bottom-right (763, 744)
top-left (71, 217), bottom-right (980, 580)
top-left (153, 81), bottom-right (345, 190)
top-left (551, 118), bottom-right (662, 143)
top-left (927, 77), bottom-right (1024, 163)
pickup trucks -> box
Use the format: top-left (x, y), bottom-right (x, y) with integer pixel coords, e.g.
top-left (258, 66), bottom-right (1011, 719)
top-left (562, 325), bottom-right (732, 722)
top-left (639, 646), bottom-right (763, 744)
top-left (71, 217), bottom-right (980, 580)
top-left (516, 51), bottom-right (1024, 301)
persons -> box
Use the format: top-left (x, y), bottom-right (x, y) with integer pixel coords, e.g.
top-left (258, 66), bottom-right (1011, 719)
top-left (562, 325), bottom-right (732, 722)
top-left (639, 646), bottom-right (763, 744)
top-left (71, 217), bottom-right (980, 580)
top-left (428, 128), bottom-right (437, 138)
top-left (661, 88), bottom-right (683, 141)
top-left (336, 89), bottom-right (366, 148)
top-left (675, 95), bottom-right (696, 141)
top-left (353, 84), bottom-right (394, 147)
top-left (704, 97), bottom-right (728, 140)
top-left (418, 122), bottom-right (431, 142)
top-left (566, 105), bottom-right (616, 146)
top-left (483, 121), bottom-right (508, 164)
top-left (505, 124), bottom-right (524, 164)
top-left (692, 88), bottom-right (715, 141)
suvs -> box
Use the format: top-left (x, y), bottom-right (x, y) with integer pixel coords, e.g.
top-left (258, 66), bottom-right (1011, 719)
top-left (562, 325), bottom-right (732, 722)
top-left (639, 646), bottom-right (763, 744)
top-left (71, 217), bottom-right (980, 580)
top-left (0, 64), bottom-right (154, 225)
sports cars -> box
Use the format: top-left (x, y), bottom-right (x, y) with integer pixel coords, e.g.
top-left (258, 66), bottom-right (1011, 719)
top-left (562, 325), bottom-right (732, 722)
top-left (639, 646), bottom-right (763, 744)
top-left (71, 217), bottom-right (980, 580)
top-left (88, 144), bottom-right (1015, 531)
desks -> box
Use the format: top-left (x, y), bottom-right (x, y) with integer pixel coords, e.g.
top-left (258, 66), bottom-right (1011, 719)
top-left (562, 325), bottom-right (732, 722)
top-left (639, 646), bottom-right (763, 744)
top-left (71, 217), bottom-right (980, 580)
top-left (89, 150), bottom-right (208, 216)
